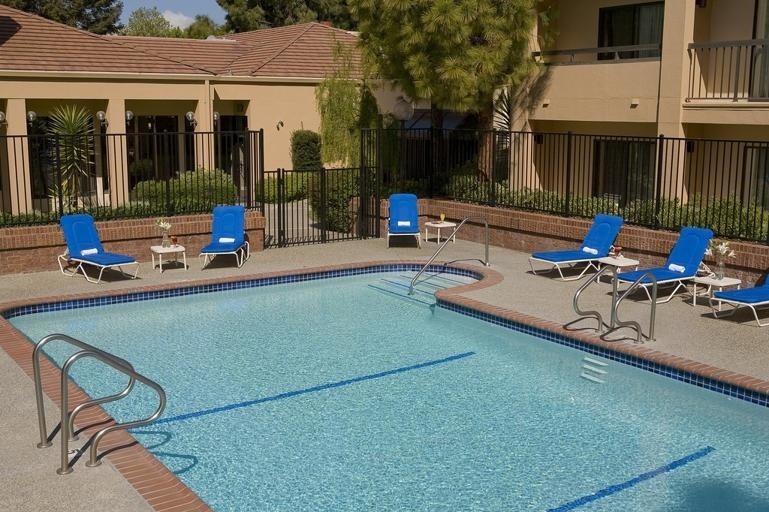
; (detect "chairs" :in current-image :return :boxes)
[617,227,715,304]
[709,272,769,327]
[386,193,422,249]
[527,213,625,281]
[57,214,139,284]
[198,205,250,270]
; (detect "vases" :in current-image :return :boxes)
[161,231,170,248]
[716,259,726,280]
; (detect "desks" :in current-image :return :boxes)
[424,221,457,245]
[150,244,187,273]
[693,277,742,312]
[597,256,640,289]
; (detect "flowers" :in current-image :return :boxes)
[703,236,737,261]
[154,214,172,232]
[607,239,623,259]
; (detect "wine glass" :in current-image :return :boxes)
[440,214,445,224]
[615,247,621,260]
[171,237,178,248]
